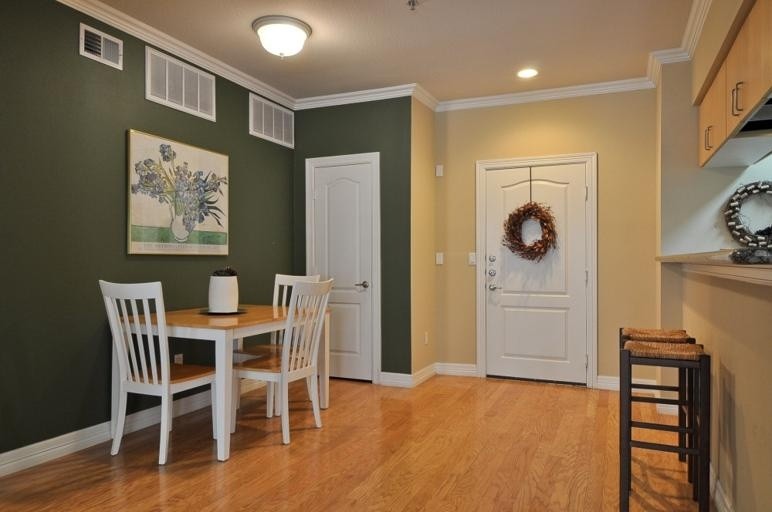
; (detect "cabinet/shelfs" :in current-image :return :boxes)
[727,6,770,145]
[697,59,724,169]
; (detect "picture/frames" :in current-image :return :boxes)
[125,126,229,259]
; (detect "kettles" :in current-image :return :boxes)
[208,265,239,314]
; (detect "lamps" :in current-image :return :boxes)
[251,14,313,61]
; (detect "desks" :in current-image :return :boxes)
[108,300,331,464]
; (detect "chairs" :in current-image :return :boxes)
[98,278,221,469]
[232,271,323,416]
[224,278,334,446]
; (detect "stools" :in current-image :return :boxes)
[621,341,715,512]
[621,329,698,489]
